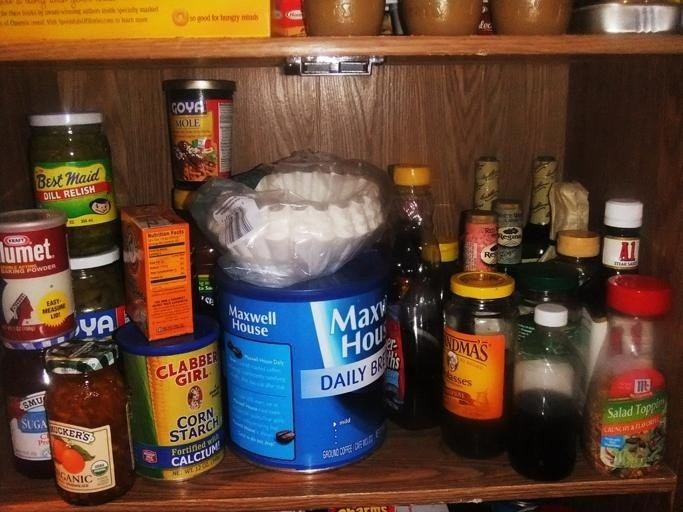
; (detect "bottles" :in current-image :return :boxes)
[560,230,602,311]
[462,207,500,271]
[582,194,645,383]
[496,198,524,271]
[589,273,671,478]
[473,157,496,208]
[123,206,195,337]
[392,164,439,437]
[516,261,570,334]
[445,272,510,460]
[425,237,458,277]
[513,303,579,480]
[545,180,586,239]
[529,152,559,240]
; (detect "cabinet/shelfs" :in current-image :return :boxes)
[0,0,680,507]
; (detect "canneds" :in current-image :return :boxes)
[174,193,213,240]
[78,247,126,331]
[31,110,114,251]
[119,312,228,481]
[210,266,389,473]
[0,207,75,342]
[46,344,134,506]
[164,79,234,189]
[194,243,218,302]
[4,344,58,482]
